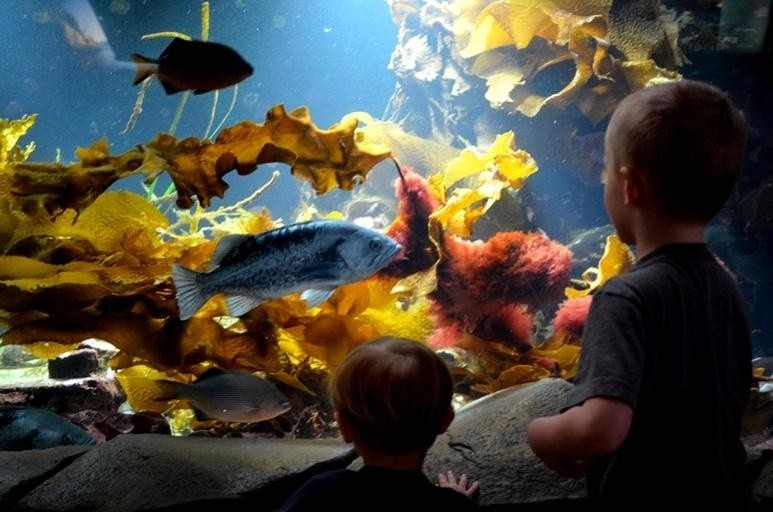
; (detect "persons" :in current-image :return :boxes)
[528,79,753,512]
[279,335,480,512]
